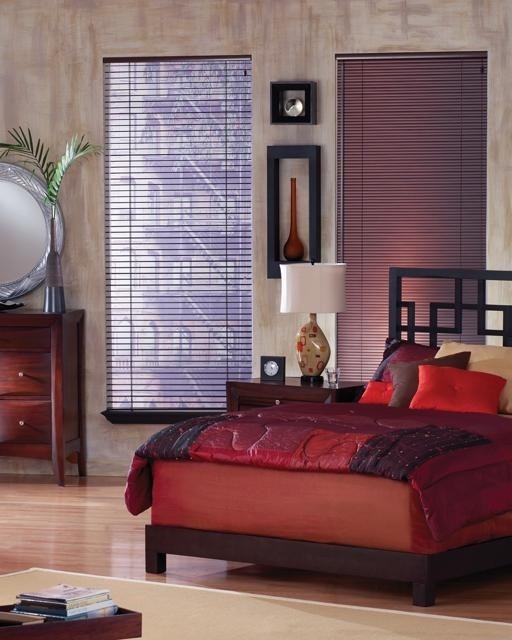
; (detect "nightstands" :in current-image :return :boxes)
[226,377,366,418]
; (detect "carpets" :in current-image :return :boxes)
[0,560,512,640]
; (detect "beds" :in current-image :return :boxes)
[121,266,512,607]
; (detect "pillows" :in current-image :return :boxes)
[358,376,396,405]
[383,348,470,410]
[372,335,439,385]
[410,357,505,420]
[432,340,512,416]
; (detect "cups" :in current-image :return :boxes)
[325,367,340,385]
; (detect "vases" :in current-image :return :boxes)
[281,175,309,263]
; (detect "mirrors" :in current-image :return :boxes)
[0,159,67,309]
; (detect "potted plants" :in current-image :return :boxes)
[0,126,112,313]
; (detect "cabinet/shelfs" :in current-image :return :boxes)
[0,305,89,484]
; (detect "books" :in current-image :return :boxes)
[0,581,120,628]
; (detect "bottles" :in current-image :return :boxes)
[283,178,304,260]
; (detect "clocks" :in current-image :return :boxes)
[259,353,288,384]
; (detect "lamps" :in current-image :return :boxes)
[276,262,347,384]
